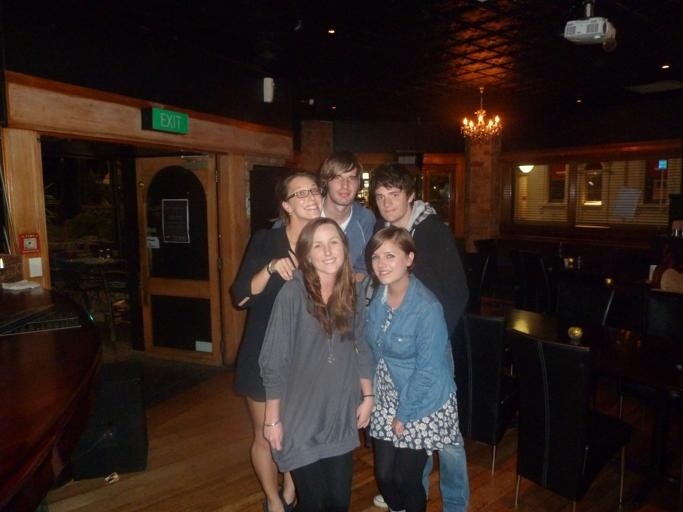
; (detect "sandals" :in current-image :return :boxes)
[281,489,297,512]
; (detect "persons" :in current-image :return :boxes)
[366,159,470,512]
[273,148,377,284]
[228,170,323,511]
[362,224,461,512]
[258,217,374,512]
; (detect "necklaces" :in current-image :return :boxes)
[325,329,337,365]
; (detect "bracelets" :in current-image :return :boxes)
[363,394,376,397]
[266,259,274,278]
[264,420,281,427]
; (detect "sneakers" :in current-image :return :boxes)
[373,493,389,508]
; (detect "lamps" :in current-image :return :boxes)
[459,87,502,144]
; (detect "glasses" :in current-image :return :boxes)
[286,187,323,201]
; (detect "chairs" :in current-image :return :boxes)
[460,236,605,332]
[505,329,631,511]
[615,289,682,507]
[449,313,522,476]
